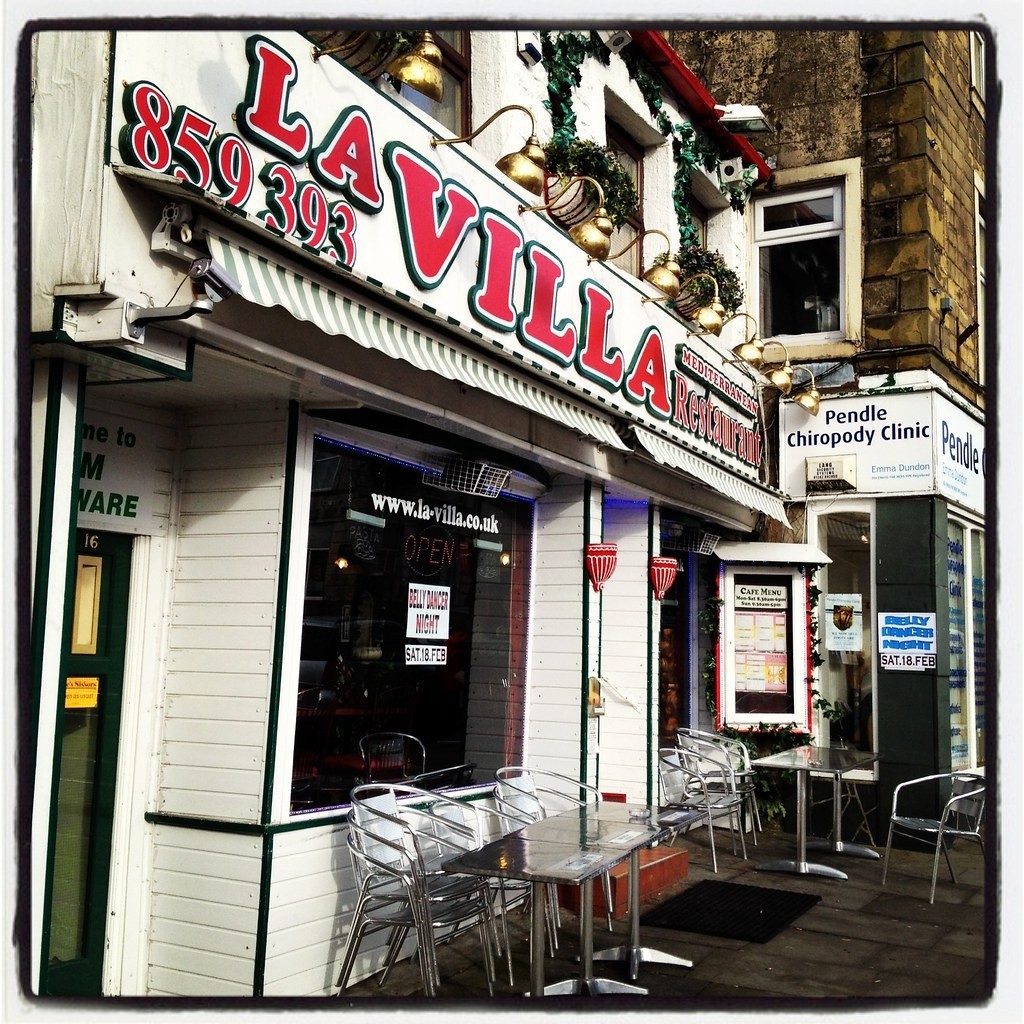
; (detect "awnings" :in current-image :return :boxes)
[148,190,802,533]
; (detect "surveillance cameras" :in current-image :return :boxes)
[188,257,242,303]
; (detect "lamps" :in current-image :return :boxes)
[430,105,547,197]
[722,340,792,395]
[313,28,444,103]
[640,273,726,337]
[687,313,764,371]
[713,103,774,135]
[752,366,820,417]
[589,229,680,296]
[518,177,612,262]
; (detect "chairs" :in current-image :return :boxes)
[333,782,496,997]
[658,747,747,874]
[289,675,476,813]
[493,766,613,957]
[674,727,764,856]
[882,772,987,904]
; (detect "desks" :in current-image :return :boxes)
[296,705,411,719]
[442,799,708,996]
[750,744,880,881]
